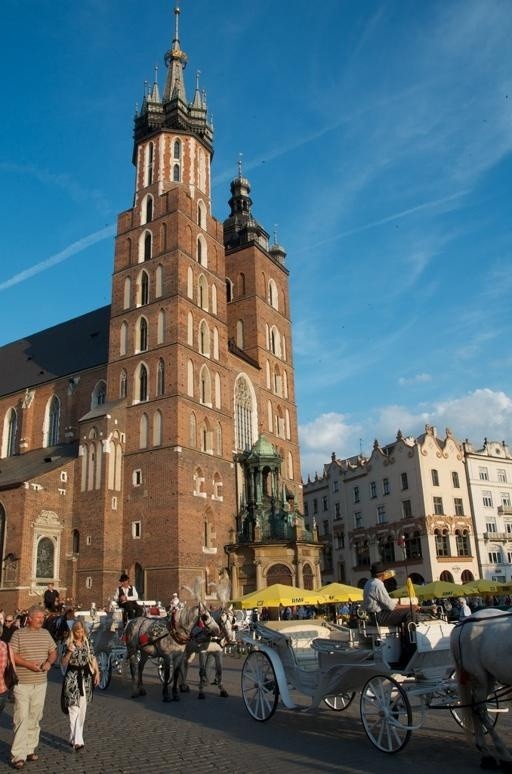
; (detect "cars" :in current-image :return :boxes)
[234,610,260,632]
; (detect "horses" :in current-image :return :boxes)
[172,603,236,699]
[119,602,221,702]
[41,607,76,669]
[450,607,512,774]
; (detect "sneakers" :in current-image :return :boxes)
[210,680,218,685]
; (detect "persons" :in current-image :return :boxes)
[115,575,143,618]
[223,615,248,654]
[57,608,77,677]
[0,610,20,713]
[363,563,421,630]
[252,604,353,623]
[59,620,100,752]
[8,605,58,768]
[43,582,62,614]
[170,593,179,607]
[458,597,471,617]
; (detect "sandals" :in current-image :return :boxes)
[26,752,39,761]
[68,741,84,753]
[13,759,25,769]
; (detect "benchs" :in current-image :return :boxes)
[109,601,163,624]
[256,618,349,658]
[74,611,108,629]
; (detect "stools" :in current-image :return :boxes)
[358,611,400,638]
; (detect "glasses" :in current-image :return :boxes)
[4,619,13,623]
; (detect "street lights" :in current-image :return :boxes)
[399,535,408,578]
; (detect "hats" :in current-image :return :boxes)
[368,561,389,575]
[119,574,130,583]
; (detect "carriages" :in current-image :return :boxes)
[60,599,238,702]
[18,602,72,648]
[236,608,510,771]
